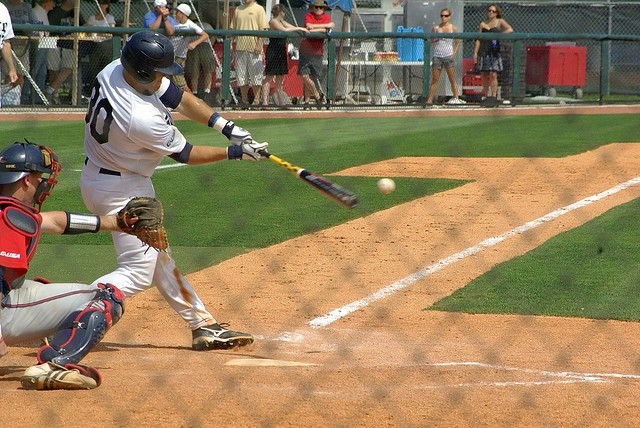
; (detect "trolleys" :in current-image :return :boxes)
[524,45,586,97]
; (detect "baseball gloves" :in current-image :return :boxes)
[115,195,168,255]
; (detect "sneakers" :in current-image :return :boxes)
[192,322,254,351]
[46,92,53,104]
[19,361,97,390]
[53,92,62,106]
[303,102,311,110]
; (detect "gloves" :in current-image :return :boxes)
[222,119,252,144]
[226,141,268,162]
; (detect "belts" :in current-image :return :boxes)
[84,157,121,177]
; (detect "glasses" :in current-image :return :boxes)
[441,14,450,17]
[488,9,497,13]
[315,6,323,9]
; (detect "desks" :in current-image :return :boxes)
[321,61,425,105]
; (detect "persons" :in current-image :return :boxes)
[143,0,176,36]
[299,0,336,110]
[423,6,461,106]
[78,30,266,348]
[189,21,215,105]
[171,4,209,96]
[82,1,118,95]
[262,0,309,111]
[472,4,515,103]
[1,0,19,84]
[23,0,58,105]
[1,0,32,87]
[48,1,86,106]
[228,0,270,110]
[0,140,138,390]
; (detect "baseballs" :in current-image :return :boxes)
[377,177,396,195]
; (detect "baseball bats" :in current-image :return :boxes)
[244,139,359,210]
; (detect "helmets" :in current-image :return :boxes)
[1,138,61,207]
[119,30,184,86]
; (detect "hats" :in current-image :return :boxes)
[309,0,328,10]
[154,0,168,8]
[170,3,192,17]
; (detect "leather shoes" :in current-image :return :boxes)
[316,93,324,110]
